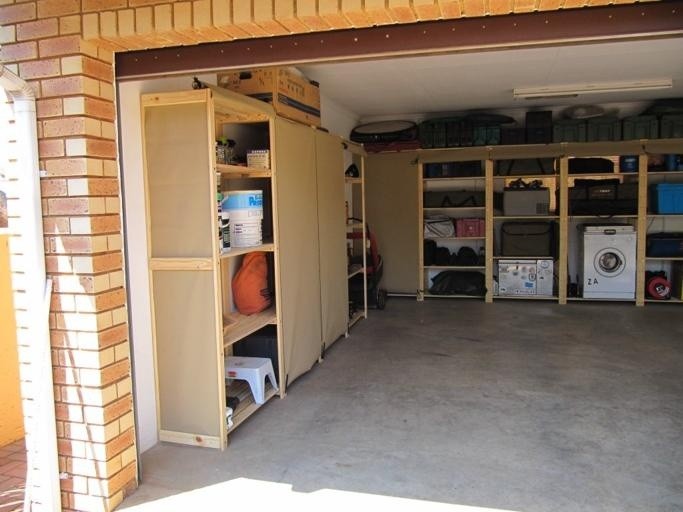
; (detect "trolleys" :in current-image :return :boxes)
[346,217,388,309]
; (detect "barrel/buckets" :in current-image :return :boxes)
[221,191,264,247]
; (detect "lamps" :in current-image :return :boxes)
[513,78,674,100]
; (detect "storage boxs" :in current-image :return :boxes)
[649,183,683,215]
[215,67,321,127]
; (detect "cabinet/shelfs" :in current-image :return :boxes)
[140,89,322,452]
[417,138,683,308]
[316,129,367,352]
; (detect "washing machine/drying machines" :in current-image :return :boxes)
[583,225,637,300]
